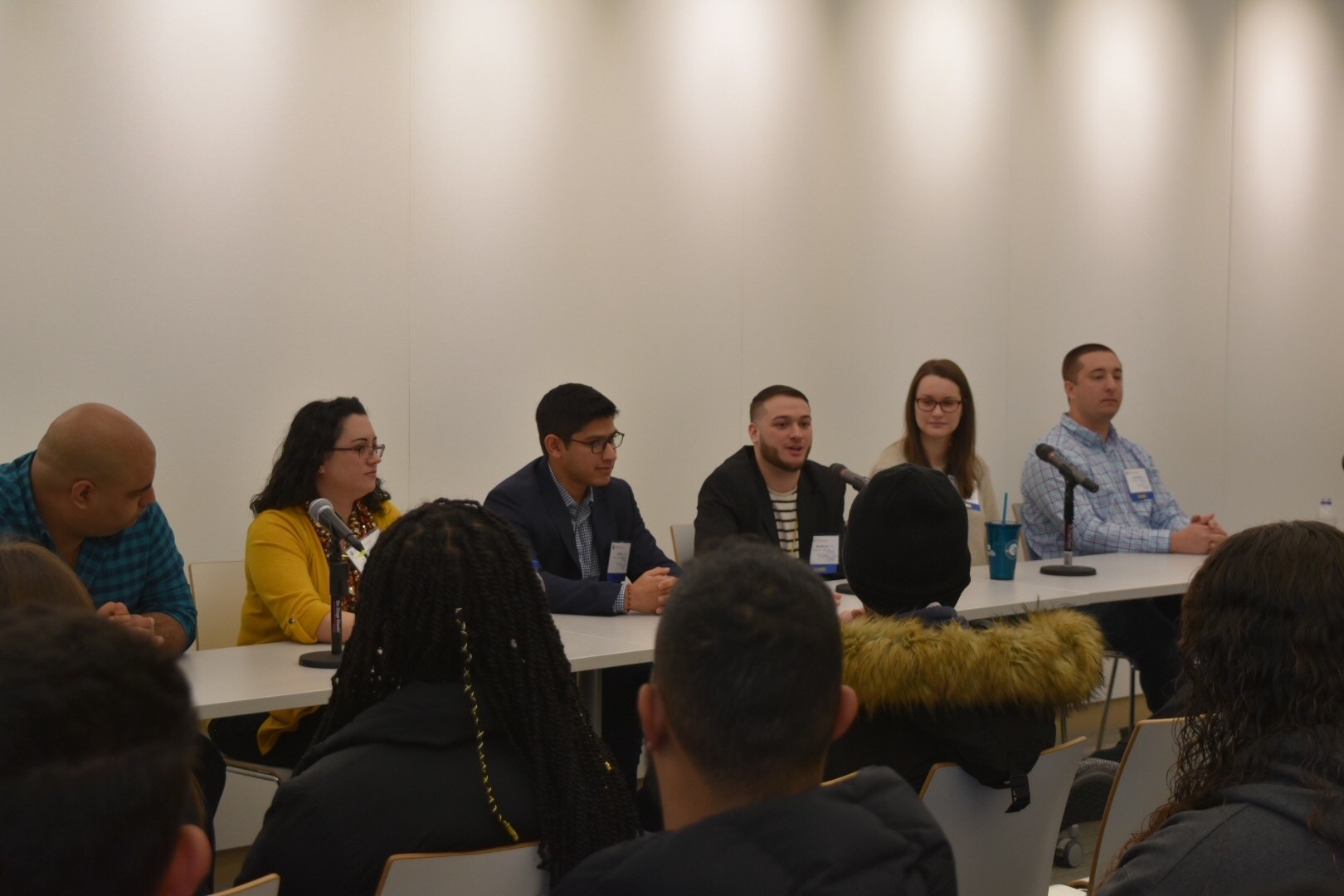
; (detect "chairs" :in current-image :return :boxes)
[917,736,1086,896]
[669,522,697,571]
[187,559,552,896]
[1069,713,1224,896]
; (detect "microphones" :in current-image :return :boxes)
[309,498,365,554]
[1036,443,1099,493]
[829,463,868,492]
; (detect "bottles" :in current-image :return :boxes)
[1315,498,1337,528]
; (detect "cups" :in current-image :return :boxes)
[985,520,1022,581]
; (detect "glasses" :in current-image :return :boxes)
[569,430,624,453]
[914,397,966,413]
[334,445,386,460]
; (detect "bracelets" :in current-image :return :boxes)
[626,584,631,613]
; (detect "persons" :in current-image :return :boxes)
[1020,344,1227,716]
[480,383,682,821]
[0,465,1344,896]
[688,384,854,582]
[870,359,1002,565]
[0,402,199,664]
[226,396,402,767]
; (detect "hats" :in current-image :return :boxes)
[843,463,971,611]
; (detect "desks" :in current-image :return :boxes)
[550,552,1208,737]
